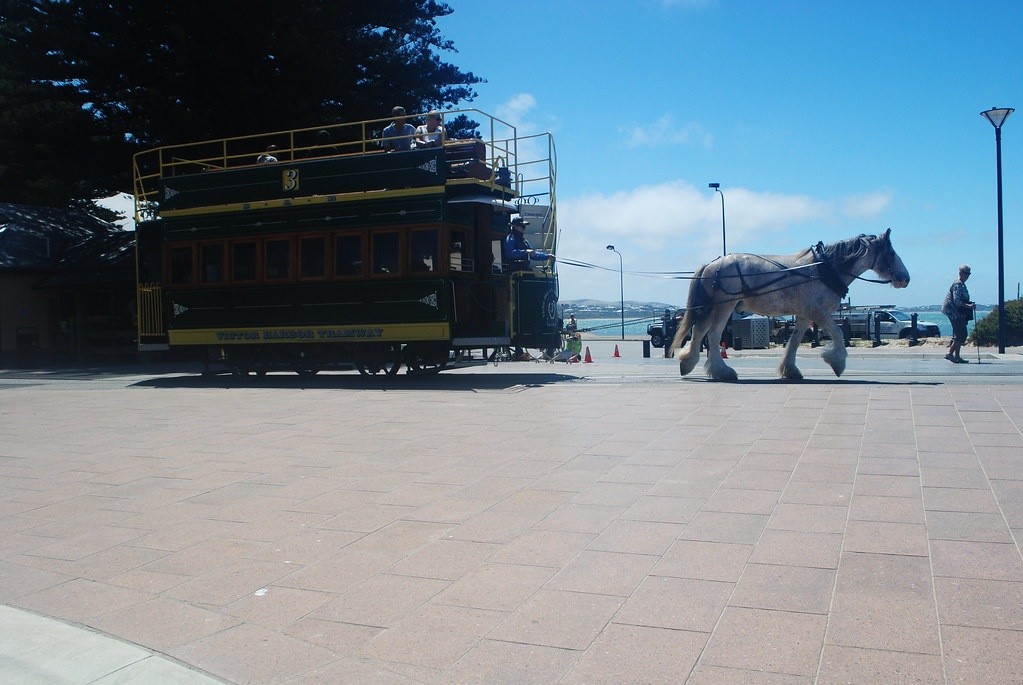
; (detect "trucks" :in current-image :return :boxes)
[808,297,940,340]
[646,308,733,346]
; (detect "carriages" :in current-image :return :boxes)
[132,108,910,380]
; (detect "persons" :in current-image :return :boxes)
[504,217,556,276]
[942,264,976,363]
[566,333,582,362]
[414,110,447,149]
[566,313,577,337]
[257,145,279,164]
[416,253,429,271]
[382,107,416,152]
[884,313,892,321]
[310,130,337,157]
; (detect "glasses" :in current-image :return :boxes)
[518,225,525,229]
[434,117,442,121]
[961,271,971,275]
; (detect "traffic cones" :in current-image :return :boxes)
[614,345,619,357]
[584,346,592,362]
[720,342,727,358]
[570,345,579,362]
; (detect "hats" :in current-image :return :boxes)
[512,217,530,225]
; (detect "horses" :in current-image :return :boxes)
[666,226,912,383]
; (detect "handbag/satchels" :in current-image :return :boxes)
[966,302,973,320]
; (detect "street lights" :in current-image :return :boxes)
[982,107,1014,354]
[708,183,726,256]
[606,245,624,339]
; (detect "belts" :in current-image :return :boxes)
[514,260,527,262]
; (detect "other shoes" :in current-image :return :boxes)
[954,357,969,363]
[944,354,959,363]
